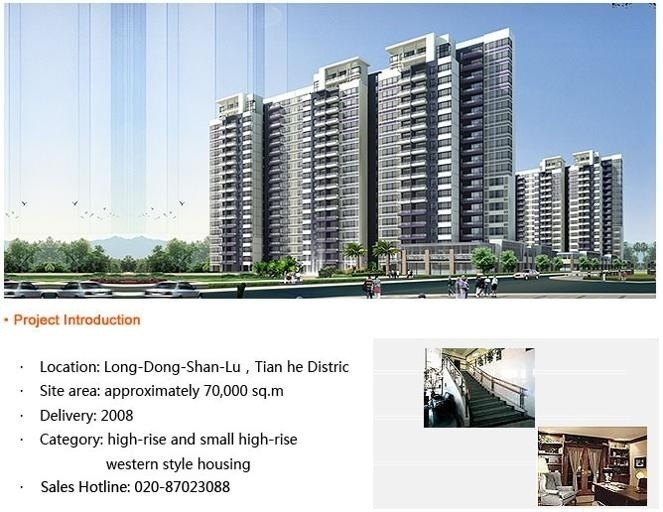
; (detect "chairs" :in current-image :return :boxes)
[638,478,647,487]
[540,472,577,506]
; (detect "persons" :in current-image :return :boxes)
[407,269,413,279]
[619,270,627,280]
[447,275,499,298]
[284,269,304,284]
[365,274,381,299]
[388,269,399,279]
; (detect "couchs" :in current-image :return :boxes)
[431,391,456,427]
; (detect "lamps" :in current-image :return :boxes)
[538,457,549,477]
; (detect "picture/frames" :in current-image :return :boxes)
[634,457,646,468]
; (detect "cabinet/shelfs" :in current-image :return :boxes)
[538,431,565,475]
[609,447,630,485]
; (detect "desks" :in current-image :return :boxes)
[591,481,647,506]
